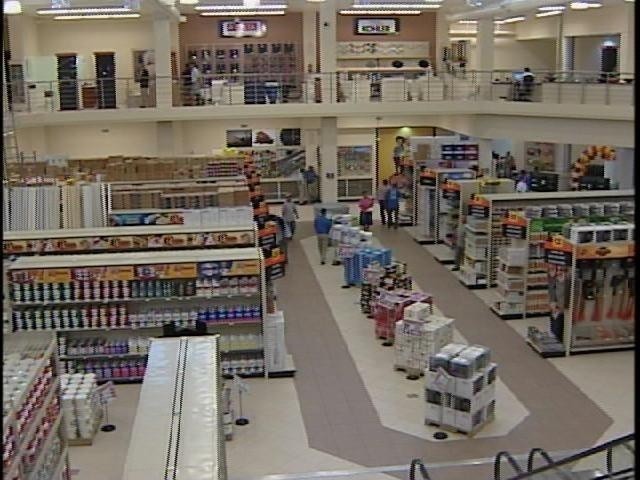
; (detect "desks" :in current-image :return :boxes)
[339,72,473,102]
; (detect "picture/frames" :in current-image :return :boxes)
[218,20,268,38]
[353,16,400,35]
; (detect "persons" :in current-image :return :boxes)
[313,208,333,266]
[384,180,411,229]
[297,168,308,206]
[179,63,194,106]
[390,136,405,177]
[501,150,531,192]
[515,66,536,90]
[377,179,393,226]
[357,190,375,233]
[281,193,300,242]
[187,60,206,106]
[302,165,321,203]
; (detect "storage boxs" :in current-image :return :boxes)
[326,215,495,437]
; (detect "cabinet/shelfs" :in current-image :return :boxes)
[2,176,267,480]
[414,166,635,358]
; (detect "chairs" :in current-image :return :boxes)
[513,72,534,100]
